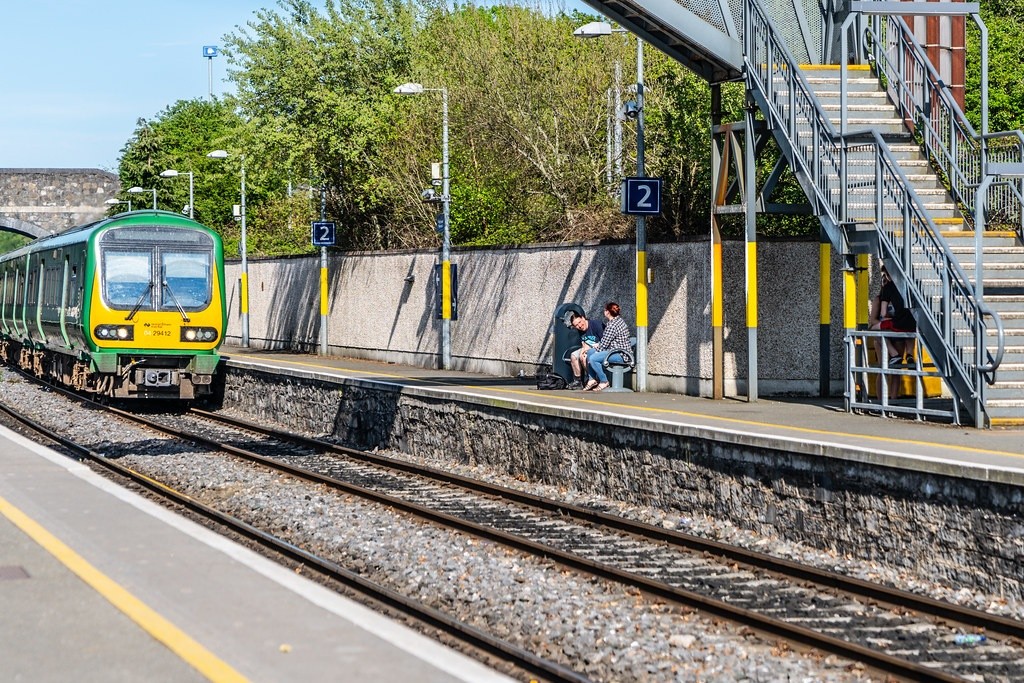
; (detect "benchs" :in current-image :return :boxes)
[562,337,636,393]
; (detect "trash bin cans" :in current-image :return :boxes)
[553,304,585,384]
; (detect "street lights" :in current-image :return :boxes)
[160,169,194,218]
[205,149,250,349]
[126,186,157,210]
[393,81,451,371]
[572,21,650,393]
[104,198,132,211]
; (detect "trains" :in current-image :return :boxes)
[0,209,230,412]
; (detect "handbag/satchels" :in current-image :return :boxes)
[534,374,567,390]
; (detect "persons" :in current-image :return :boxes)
[565,313,606,389]
[582,303,634,391]
[867,266,916,400]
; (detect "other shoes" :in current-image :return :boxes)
[905,355,915,370]
[889,354,903,365]
[583,381,597,390]
[567,381,583,389]
[593,381,610,392]
